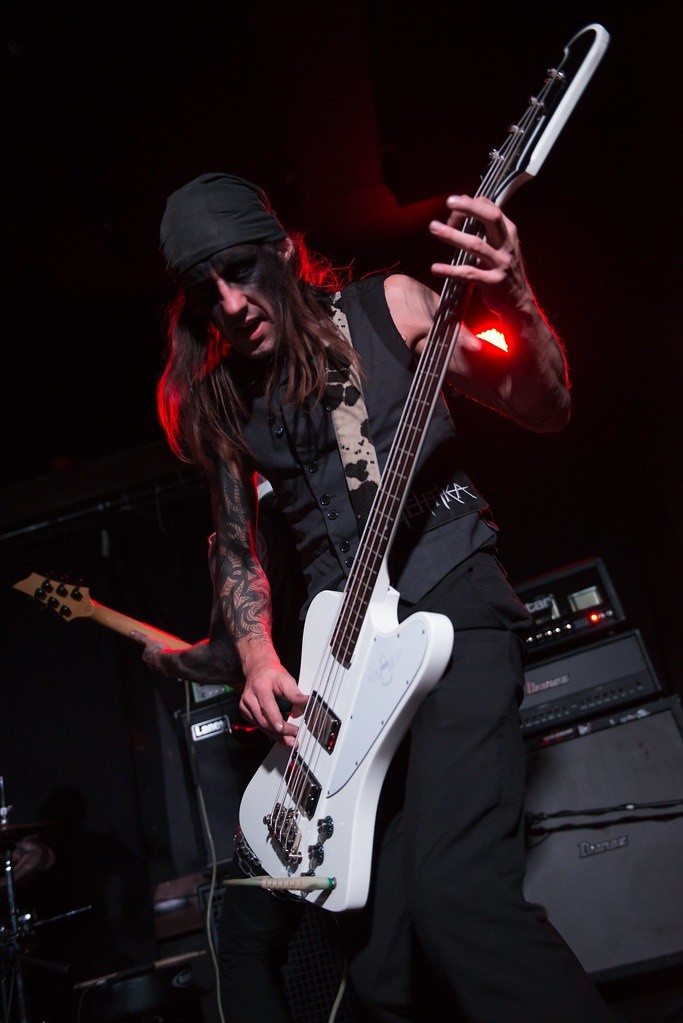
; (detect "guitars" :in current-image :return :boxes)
[238,20,611,916]
[13,570,193,651]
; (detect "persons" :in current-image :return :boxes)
[0,775,55,1023]
[142,519,307,1023]
[157,173,621,1023]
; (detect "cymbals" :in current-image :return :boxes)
[0,819,69,847]
